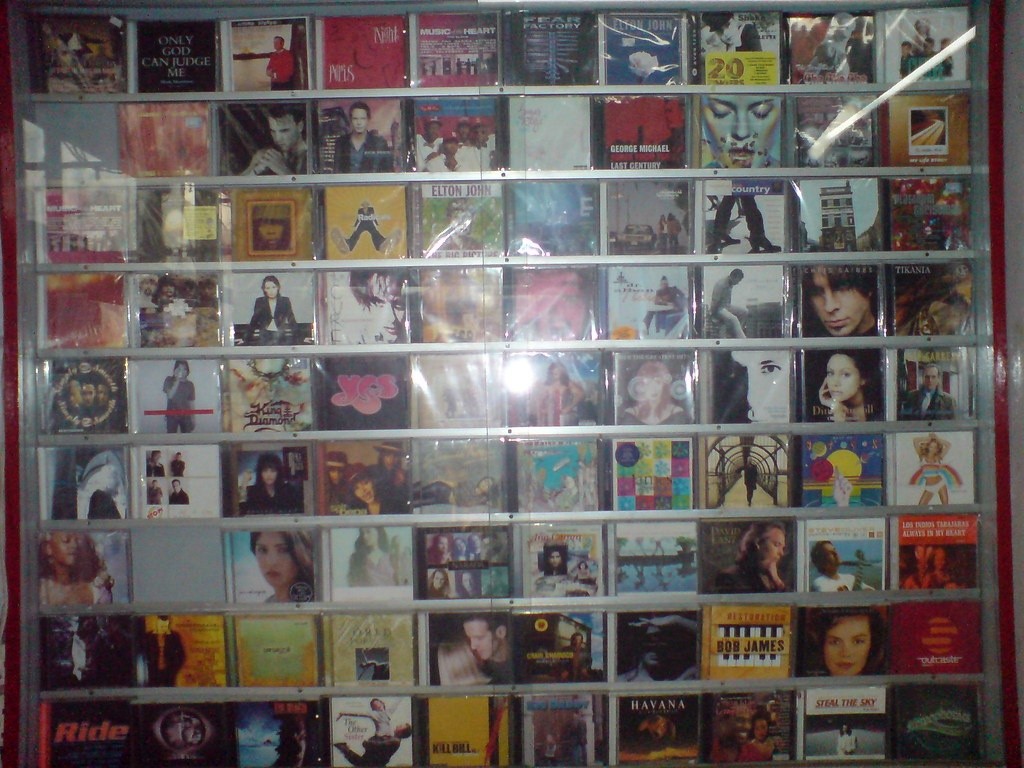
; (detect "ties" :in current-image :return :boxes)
[921,393,930,418]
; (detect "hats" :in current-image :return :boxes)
[325,452,346,471]
[372,440,404,455]
[339,464,373,493]
[252,206,288,221]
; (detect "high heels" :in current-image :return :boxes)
[749,231,781,253]
[713,230,740,246]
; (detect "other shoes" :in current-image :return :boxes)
[380,229,402,256]
[330,228,349,254]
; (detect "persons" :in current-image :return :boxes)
[14,7,977,766]
